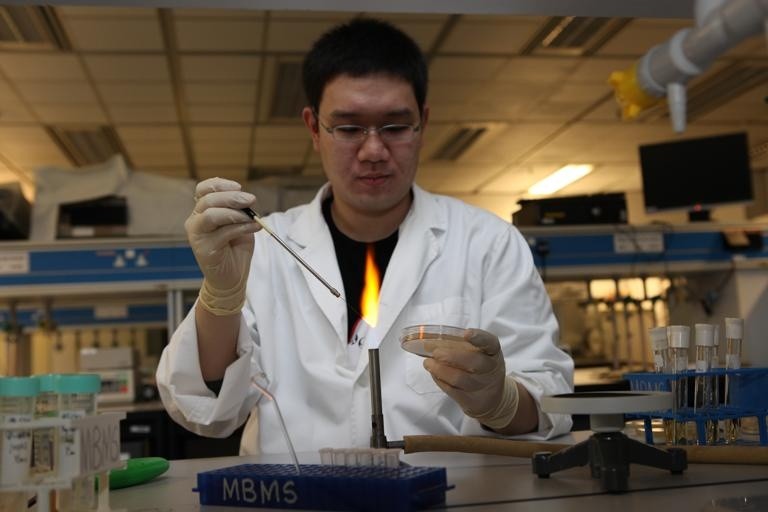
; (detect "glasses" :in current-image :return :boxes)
[316,119,423,145]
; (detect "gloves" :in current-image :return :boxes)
[421,326,520,431]
[184,178,262,316]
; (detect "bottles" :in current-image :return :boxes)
[651,319,744,451]
[318,448,399,470]
[0,373,112,512]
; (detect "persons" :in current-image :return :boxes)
[154,16,574,460]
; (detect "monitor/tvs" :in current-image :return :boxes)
[638,131,754,214]
